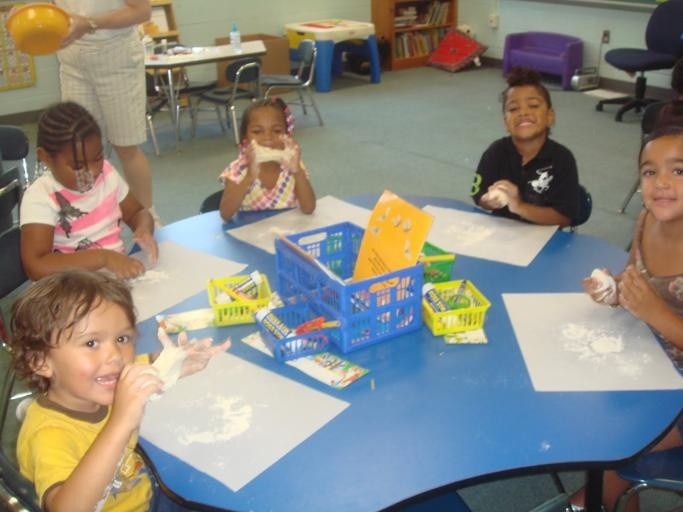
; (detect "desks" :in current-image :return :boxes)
[126,193,680,512]
[282,17,381,93]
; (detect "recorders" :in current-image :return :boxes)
[569,67,600,90]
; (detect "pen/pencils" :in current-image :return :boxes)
[310,320,341,329]
[418,254,456,262]
[295,317,325,334]
[454,279,467,303]
[211,278,258,312]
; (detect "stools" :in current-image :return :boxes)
[613,447,681,512]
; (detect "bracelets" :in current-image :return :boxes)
[88,17,97,35]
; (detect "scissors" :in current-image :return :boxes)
[446,295,471,310]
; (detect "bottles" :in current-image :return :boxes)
[144,36,193,61]
[217,271,451,349]
[229,21,242,51]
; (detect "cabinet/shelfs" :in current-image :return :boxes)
[145,2,188,108]
[370,0,457,71]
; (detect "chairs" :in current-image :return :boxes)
[0,449,40,512]
[595,0,682,122]
[144,32,323,156]
[0,222,30,434]
[614,100,683,217]
[503,30,582,90]
[197,189,222,214]
[1,123,31,186]
[0,165,23,226]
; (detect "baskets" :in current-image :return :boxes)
[273,222,425,355]
[206,272,273,327]
[420,239,456,282]
[421,278,491,337]
[253,304,333,361]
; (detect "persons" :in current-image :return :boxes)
[10,266,232,512]
[471,65,578,229]
[217,96,316,221]
[18,101,158,281]
[566,100,683,512]
[53,0,161,227]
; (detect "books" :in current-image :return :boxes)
[394,0,450,58]
